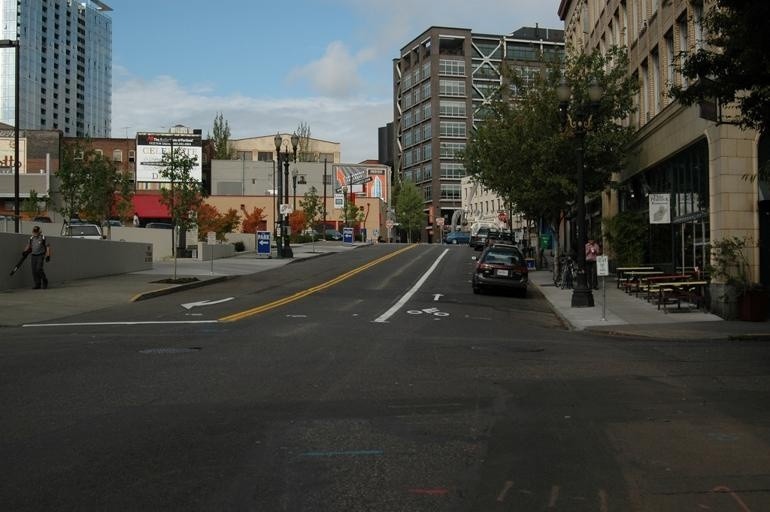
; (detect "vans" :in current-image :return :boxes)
[145,222,175,229]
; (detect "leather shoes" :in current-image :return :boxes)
[43,279,48,288]
[33,285,41,288]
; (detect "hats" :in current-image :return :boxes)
[32,226,42,234]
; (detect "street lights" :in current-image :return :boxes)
[0,40,20,233]
[554,73,604,308]
[274,131,299,258]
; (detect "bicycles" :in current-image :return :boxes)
[532,247,548,268]
[554,256,575,290]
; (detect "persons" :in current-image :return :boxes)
[22,226,51,289]
[133,212,139,228]
[585,236,600,290]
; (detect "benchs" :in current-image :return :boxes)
[616,265,710,314]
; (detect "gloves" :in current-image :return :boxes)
[45,256,50,262]
[22,251,27,256]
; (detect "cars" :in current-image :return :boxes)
[442,227,528,296]
[33,215,122,240]
[300,229,343,241]
